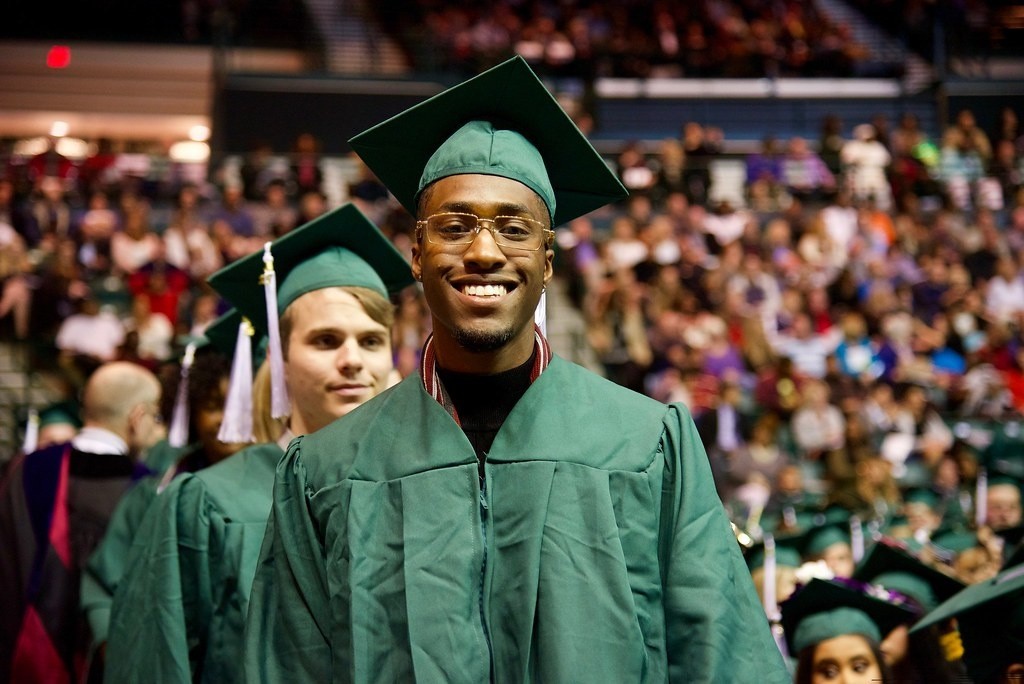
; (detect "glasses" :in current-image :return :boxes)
[414,211,557,251]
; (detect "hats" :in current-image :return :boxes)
[849,540,968,613]
[907,563,1024,634]
[205,202,415,418]
[728,409,1023,575]
[204,307,264,446]
[348,54,629,229]
[780,578,916,656]
[24,397,79,454]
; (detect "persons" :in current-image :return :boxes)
[0,361,166,684]
[103,202,417,684]
[419,0,872,57]
[82,306,289,664]
[1,109,1023,610]
[777,577,915,684]
[238,57,793,684]
[847,539,966,684]
[907,576,1023,684]
